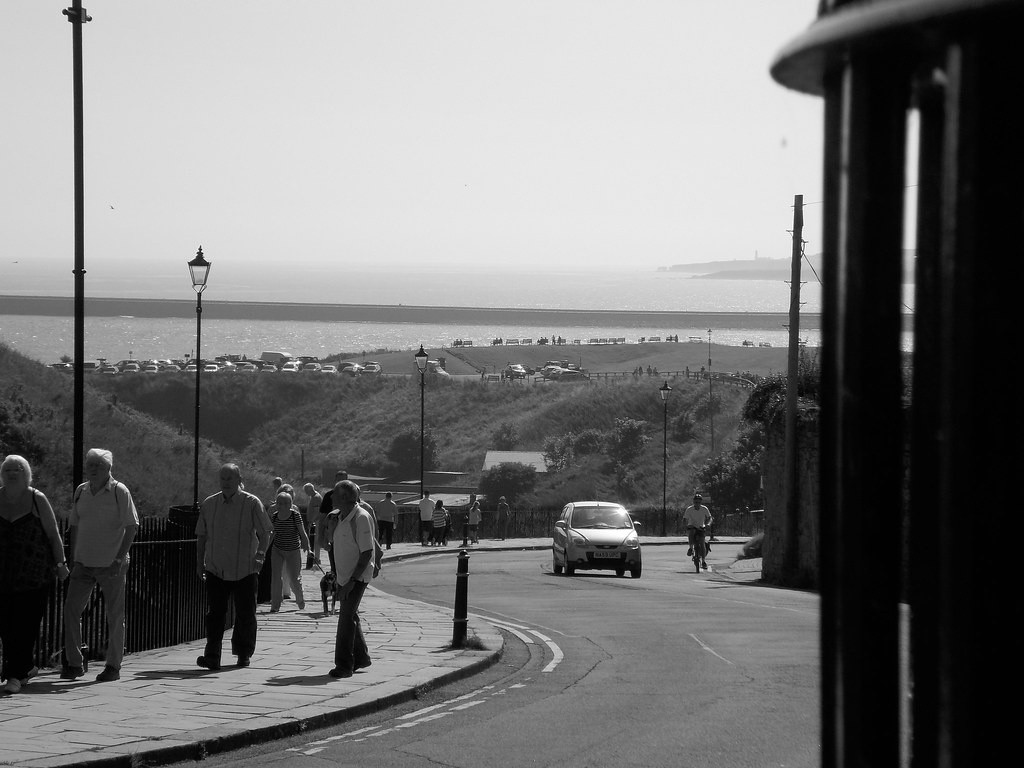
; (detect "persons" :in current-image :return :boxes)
[0,455,69,693]
[195,461,511,677]
[61,448,140,682]
[682,494,712,569]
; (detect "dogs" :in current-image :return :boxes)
[319,571,340,617]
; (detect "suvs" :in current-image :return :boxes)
[337,361,382,377]
[504,364,535,379]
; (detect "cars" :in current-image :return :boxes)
[242,364,258,373]
[281,360,303,373]
[552,501,642,578]
[321,365,337,376]
[260,365,278,373]
[204,365,220,372]
[234,351,320,367]
[186,365,198,372]
[302,363,321,373]
[53,359,181,375]
[536,360,579,377]
[223,365,238,373]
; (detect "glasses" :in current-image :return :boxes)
[693,494,700,498]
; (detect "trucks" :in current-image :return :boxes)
[413,361,449,378]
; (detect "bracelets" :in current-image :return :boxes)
[255,559,263,564]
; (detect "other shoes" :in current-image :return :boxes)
[298,601,305,610]
[96,665,120,681]
[59,667,84,680]
[270,609,280,613]
[329,665,353,678]
[702,562,708,569]
[237,655,250,666]
[687,548,694,556]
[353,653,372,674]
[197,655,221,670]
[5,666,38,692]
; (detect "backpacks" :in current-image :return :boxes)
[350,512,383,578]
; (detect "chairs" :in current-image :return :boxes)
[602,510,618,527]
[573,510,588,526]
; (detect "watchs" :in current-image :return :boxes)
[114,558,123,563]
[349,576,356,582]
[57,561,68,567]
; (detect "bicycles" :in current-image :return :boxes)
[685,525,707,573]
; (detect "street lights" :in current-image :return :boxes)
[659,381,672,535]
[707,328,712,370]
[186,246,211,535]
[414,344,429,499]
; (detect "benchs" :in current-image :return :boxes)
[586,337,625,345]
[572,339,581,345]
[741,341,772,348]
[647,337,661,342]
[665,337,675,342]
[689,336,703,342]
[638,336,645,343]
[537,339,548,346]
[520,339,532,345]
[798,341,807,347]
[493,340,503,346]
[555,339,567,345]
[506,338,519,346]
[485,374,500,382]
[454,341,472,347]
